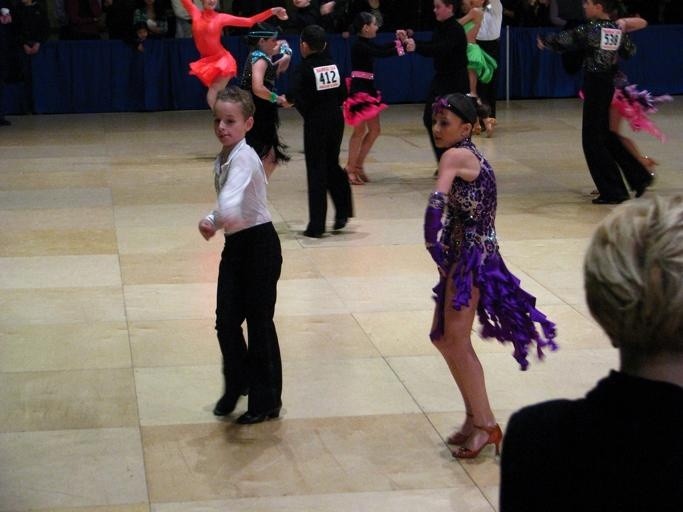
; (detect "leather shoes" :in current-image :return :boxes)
[591,177,652,204]
[234,400,282,424]
[214,385,251,415]
[300,218,348,237]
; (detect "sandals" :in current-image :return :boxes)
[344,163,369,185]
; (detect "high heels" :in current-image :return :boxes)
[446,411,503,460]
[473,117,496,137]
[643,155,659,180]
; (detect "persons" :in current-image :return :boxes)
[180,0,503,186]
[199,85,283,425]
[499,195,683,512]
[538,1,676,205]
[422,93,502,459]
[286,24,353,237]
[1,1,12,126]
[13,2,190,54]
